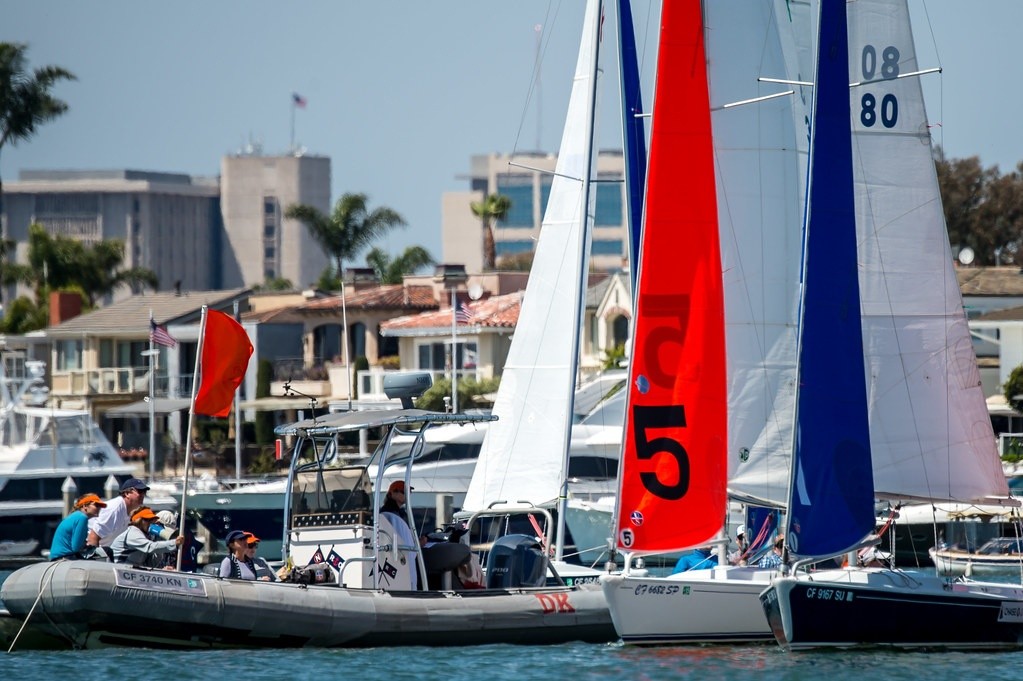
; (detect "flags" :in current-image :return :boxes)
[194,308,254,417]
[294,96,306,108]
[456,297,472,324]
[150,322,176,348]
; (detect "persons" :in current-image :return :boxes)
[759,534,784,569]
[219,530,282,582]
[86,478,150,548]
[382,481,427,546]
[727,525,744,562]
[49,493,107,560]
[672,546,718,574]
[109,506,184,570]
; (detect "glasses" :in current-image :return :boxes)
[737,534,744,539]
[396,489,411,495]
[86,503,101,509]
[773,543,782,549]
[246,543,259,548]
[132,489,147,495]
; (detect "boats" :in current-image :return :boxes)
[561,480,756,567]
[169,283,787,577]
[929,511,1023,577]
[0,538,39,556]
[870,496,1023,564]
[0,343,142,553]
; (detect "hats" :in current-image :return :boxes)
[225,531,252,546]
[120,479,150,492]
[242,531,260,543]
[736,524,746,534]
[774,534,785,544]
[389,480,414,491]
[132,509,160,521]
[75,494,107,508]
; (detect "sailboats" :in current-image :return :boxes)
[0,0,1023,652]
